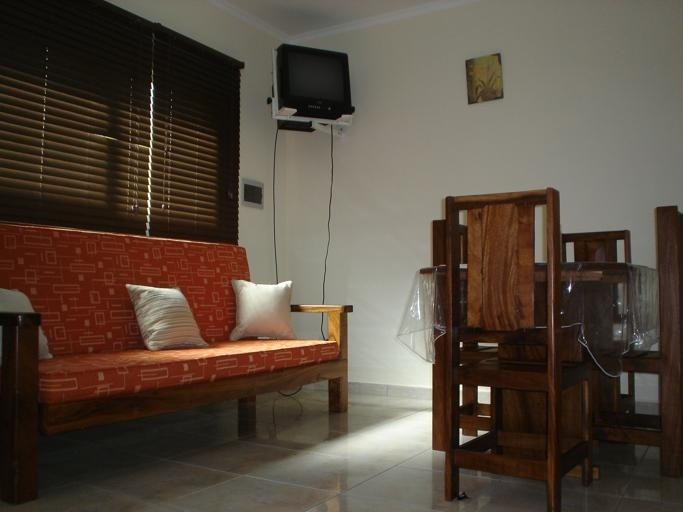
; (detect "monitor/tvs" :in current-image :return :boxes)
[276,43,355,119]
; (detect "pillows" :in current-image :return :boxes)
[227,275,298,342]
[122,278,209,355]
[0,283,54,364]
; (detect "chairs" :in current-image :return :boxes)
[544,224,631,414]
[428,214,495,453]
[439,179,598,511]
[579,198,682,486]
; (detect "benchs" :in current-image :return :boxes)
[0,216,353,507]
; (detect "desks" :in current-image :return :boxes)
[414,261,661,486]
